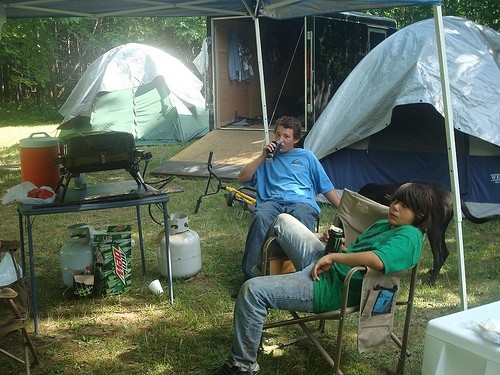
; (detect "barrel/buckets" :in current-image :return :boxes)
[20,132,61,191]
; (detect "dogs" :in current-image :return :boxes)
[338,182,455,286]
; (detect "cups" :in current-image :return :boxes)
[148,279,164,295]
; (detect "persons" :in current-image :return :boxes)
[216,182,437,375]
[230,115,341,300]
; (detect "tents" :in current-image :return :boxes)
[57,43,209,145]
[0,1,500,314]
[291,15,500,225]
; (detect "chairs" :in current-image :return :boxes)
[256,189,426,375]
[0,239,40,375]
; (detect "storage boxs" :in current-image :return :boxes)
[63,268,95,299]
[421,300,500,375]
[92,225,133,297]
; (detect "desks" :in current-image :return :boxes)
[17,181,173,334]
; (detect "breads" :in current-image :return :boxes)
[28,188,53,198]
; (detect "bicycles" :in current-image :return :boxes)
[193,150,258,216]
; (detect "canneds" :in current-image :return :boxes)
[266,140,281,161]
[324,227,343,255]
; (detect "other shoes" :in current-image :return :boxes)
[216,359,260,375]
[230,283,243,302]
